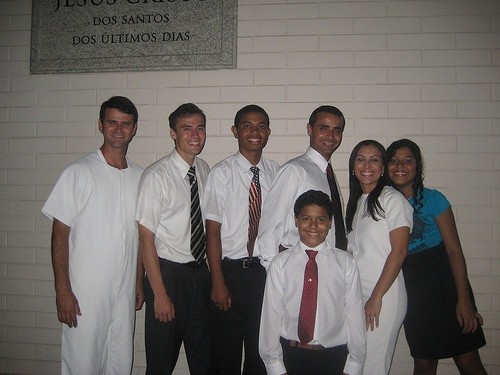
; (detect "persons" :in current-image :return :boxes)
[202,104,282,375]
[41,96,147,375]
[385,139,491,375]
[258,189,367,375]
[342,140,413,375]
[260,105,347,273]
[134,102,212,375]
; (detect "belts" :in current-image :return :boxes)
[222,258,258,270]
[286,340,345,353]
[158,256,207,267]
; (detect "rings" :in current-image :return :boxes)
[369,318,374,321]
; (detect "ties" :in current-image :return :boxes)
[326,163,348,251]
[246,166,262,261]
[297,250,319,345]
[187,167,207,265]
